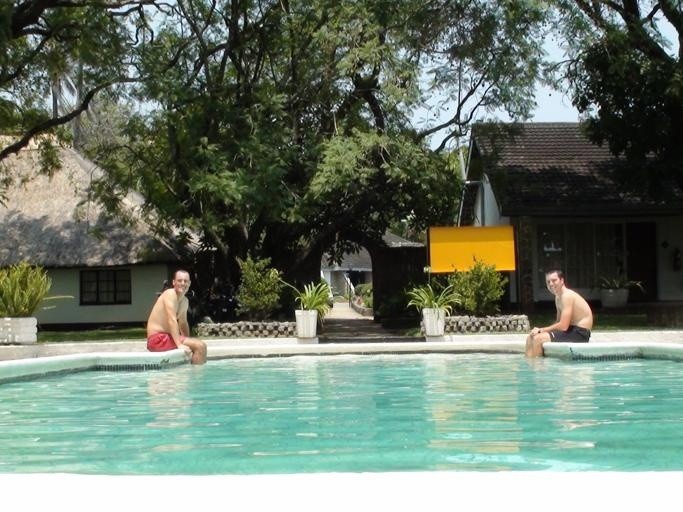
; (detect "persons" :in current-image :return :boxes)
[147,270,208,366]
[525,268,594,358]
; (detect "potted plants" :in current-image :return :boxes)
[405,282,463,337]
[598,267,647,309]
[275,268,330,339]
[0,258,76,344]
